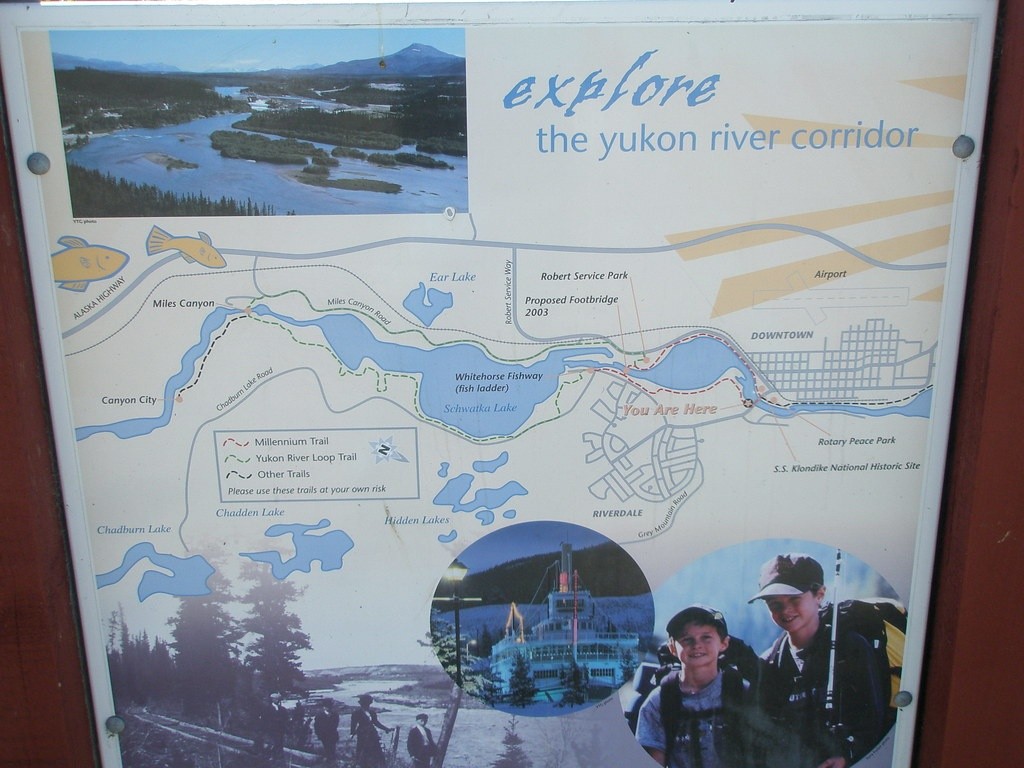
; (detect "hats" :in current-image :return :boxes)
[666,605,725,636]
[358,695,373,705]
[748,553,825,602]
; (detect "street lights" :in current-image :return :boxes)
[465,639,477,667]
[446,558,470,690]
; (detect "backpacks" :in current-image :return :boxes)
[771,600,891,719]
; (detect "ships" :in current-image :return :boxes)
[488,540,641,699]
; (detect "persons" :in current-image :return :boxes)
[637,606,751,768]
[751,552,908,768]
[260,693,441,768]
